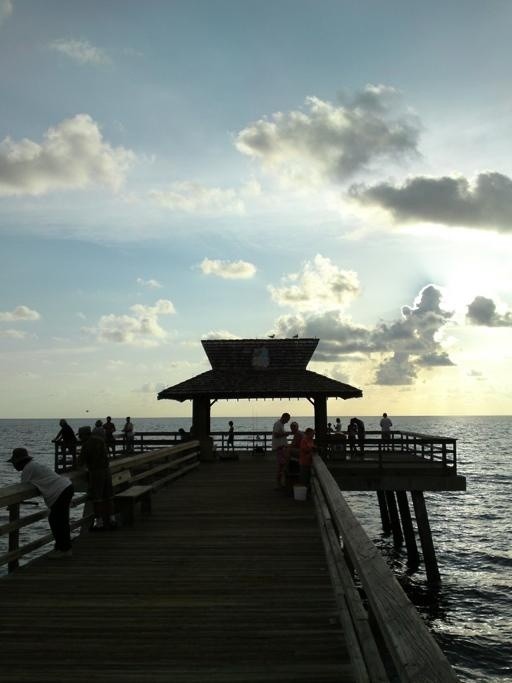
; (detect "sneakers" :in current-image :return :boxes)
[46,547,73,559]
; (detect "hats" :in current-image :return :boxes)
[6,448,35,463]
[75,426,93,436]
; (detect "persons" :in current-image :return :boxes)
[179,427,190,441]
[92,419,105,440]
[224,419,235,452]
[378,412,393,451]
[73,426,120,533]
[53,418,79,462]
[7,447,77,560]
[102,416,117,459]
[268,409,368,494]
[122,417,135,453]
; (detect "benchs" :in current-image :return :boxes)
[111,469,153,523]
